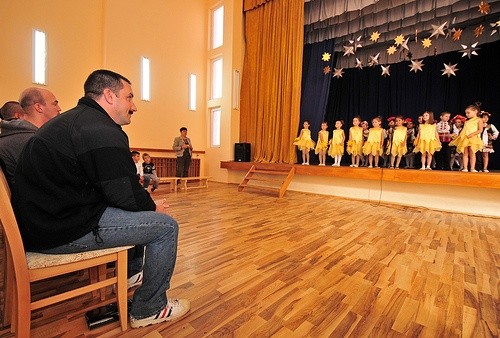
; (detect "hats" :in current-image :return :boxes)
[450,114,467,124]
[478,111,491,116]
[405,118,415,123]
[361,121,368,126]
[387,117,396,122]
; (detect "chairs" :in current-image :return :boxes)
[0,166,135,338]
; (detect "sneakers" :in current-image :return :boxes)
[129,298,190,329]
[112,270,144,294]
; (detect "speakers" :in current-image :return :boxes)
[235,143,251,162]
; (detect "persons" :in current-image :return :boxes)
[449,103,484,172]
[0,87,61,181]
[479,112,499,172]
[131,151,159,192]
[413,111,442,170]
[363,116,396,168]
[434,111,467,172]
[328,120,345,166]
[347,116,369,167]
[173,127,193,186]
[11,69,190,327]
[315,121,329,166]
[293,121,315,165]
[387,116,417,169]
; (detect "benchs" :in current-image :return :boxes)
[153,176,210,194]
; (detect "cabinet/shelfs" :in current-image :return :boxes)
[152,158,201,179]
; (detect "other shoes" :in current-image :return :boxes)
[479,169,489,173]
[368,165,380,169]
[405,165,415,168]
[450,165,463,171]
[302,163,309,166]
[361,164,368,166]
[332,163,340,167]
[151,188,155,192]
[420,167,431,170]
[350,164,358,168]
[431,161,436,168]
[389,166,399,170]
[462,168,478,173]
[319,164,325,167]
